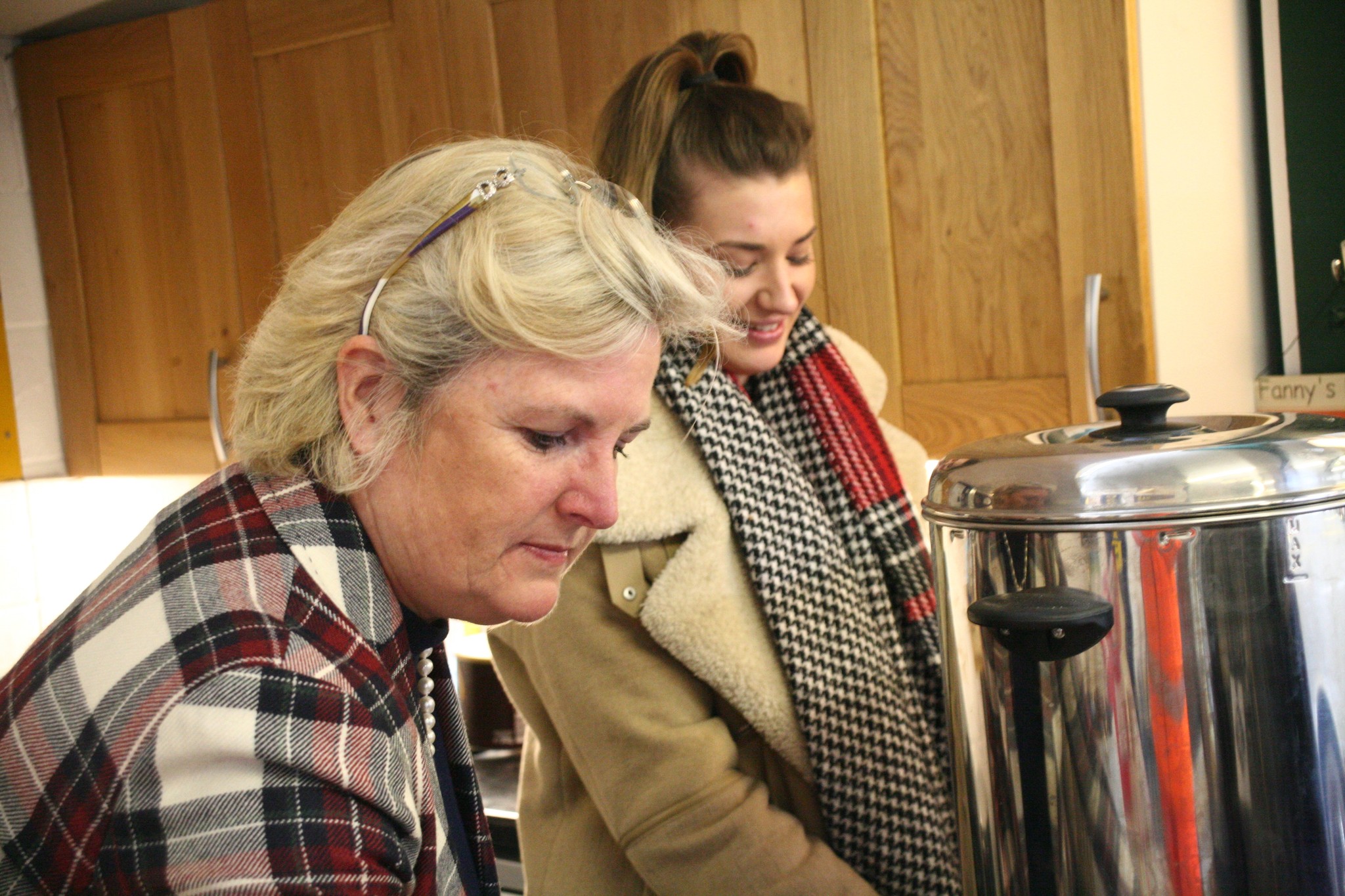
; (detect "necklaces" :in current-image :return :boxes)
[1003,532,1029,591]
[417,648,436,760]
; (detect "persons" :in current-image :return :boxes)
[3,138,751,896]
[490,31,958,896]
[953,486,1158,896]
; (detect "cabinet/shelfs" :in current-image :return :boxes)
[6,2,1160,479]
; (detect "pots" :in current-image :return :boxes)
[919,384,1345,896]
